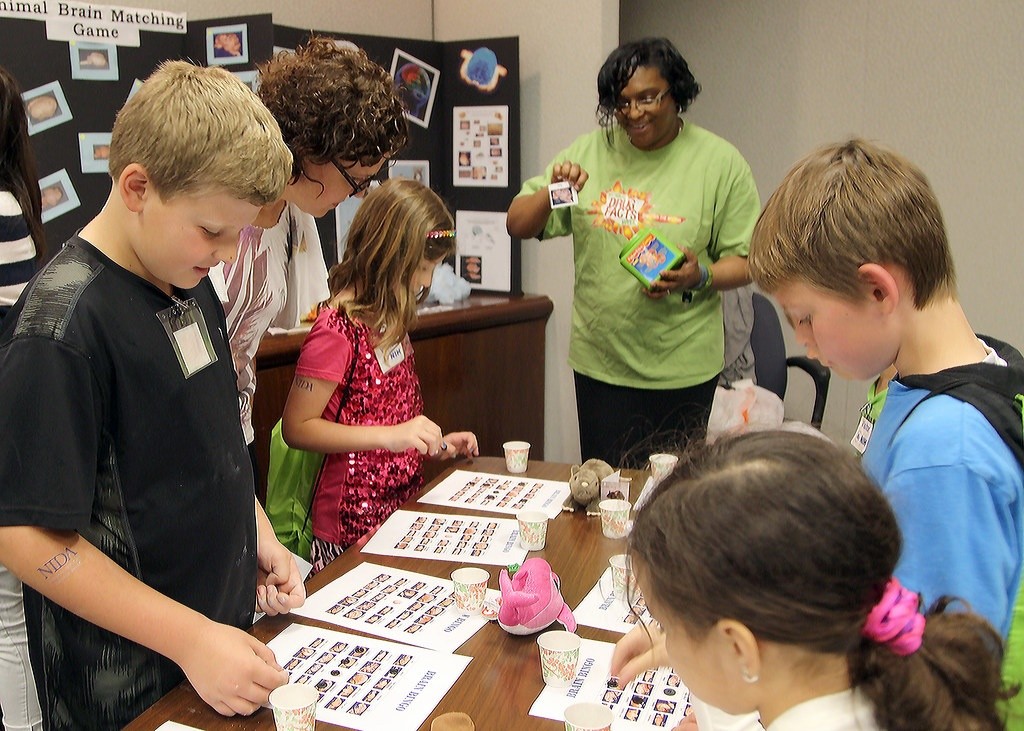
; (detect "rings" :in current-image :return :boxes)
[666,288,671,296]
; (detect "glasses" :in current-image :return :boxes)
[330,160,376,198]
[614,88,671,115]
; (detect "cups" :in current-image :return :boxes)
[503,441,531,473]
[537,631,581,687]
[608,554,641,601]
[649,454,678,485]
[451,567,491,616]
[564,702,615,731]
[599,499,632,539]
[268,683,318,731]
[516,510,548,551]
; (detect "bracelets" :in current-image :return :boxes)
[690,262,713,292]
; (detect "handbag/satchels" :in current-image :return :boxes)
[706,377,784,444]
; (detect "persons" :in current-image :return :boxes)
[0,58,309,731]
[609,428,1024,730]
[279,176,479,586]
[206,39,411,443]
[746,134,1024,731]
[505,38,761,472]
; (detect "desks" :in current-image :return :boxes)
[251,295,554,509]
[120,451,654,731]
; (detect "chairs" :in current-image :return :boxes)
[749,295,833,430]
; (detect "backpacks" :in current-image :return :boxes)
[265,324,360,561]
